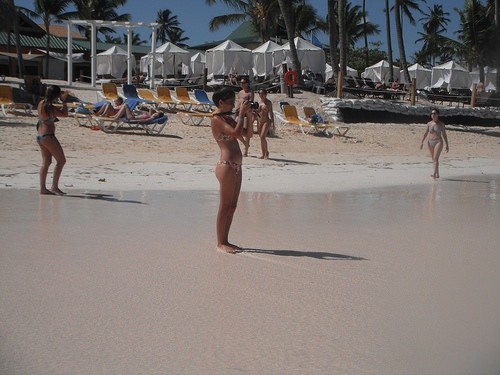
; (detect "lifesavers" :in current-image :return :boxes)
[284,71,298,86]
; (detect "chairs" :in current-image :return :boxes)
[223,71,472,136]
[0,71,217,137]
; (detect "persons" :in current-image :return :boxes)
[375,81,383,90]
[36,85,69,194]
[228,74,238,85]
[287,68,294,98]
[478,80,483,92]
[133,73,147,83]
[420,108,449,179]
[392,79,400,98]
[234,75,275,159]
[211,87,254,253]
[93,96,164,121]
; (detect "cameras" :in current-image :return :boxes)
[242,99,259,110]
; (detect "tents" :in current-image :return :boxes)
[140,37,357,81]
[361,60,497,92]
[96,45,136,78]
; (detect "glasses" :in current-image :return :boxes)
[239,81,248,84]
[431,113,437,115]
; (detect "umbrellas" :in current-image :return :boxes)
[6,11,46,54]
